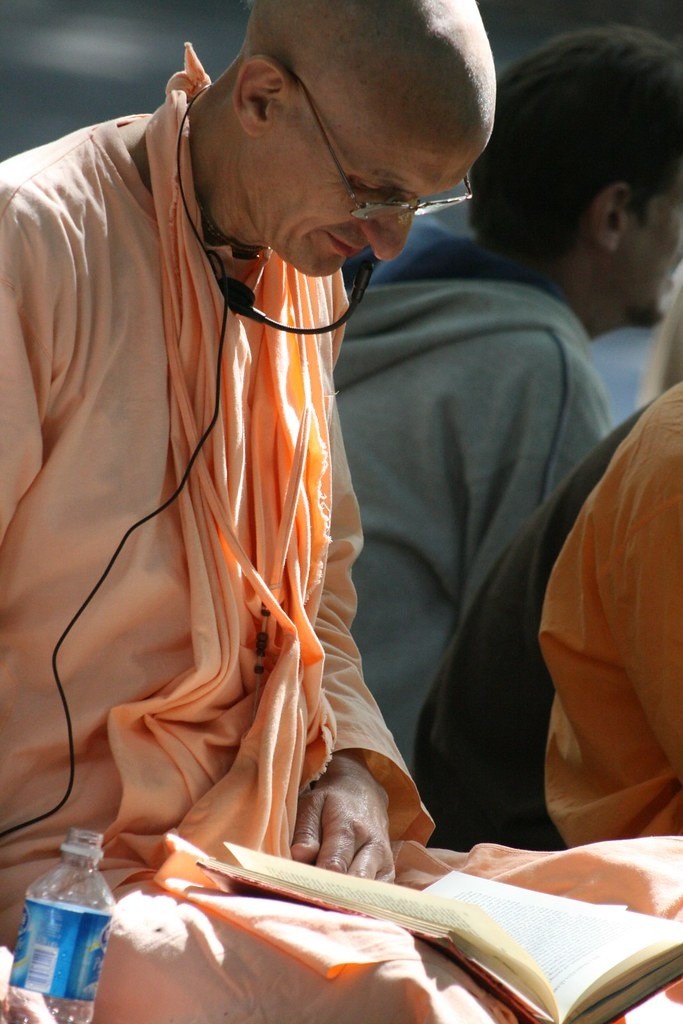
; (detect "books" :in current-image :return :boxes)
[197,839,683,1024]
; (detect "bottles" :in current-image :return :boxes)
[7,828,117,1024]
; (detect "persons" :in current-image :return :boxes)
[0,0,683,1024]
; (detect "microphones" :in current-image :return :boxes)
[219,259,374,334]
